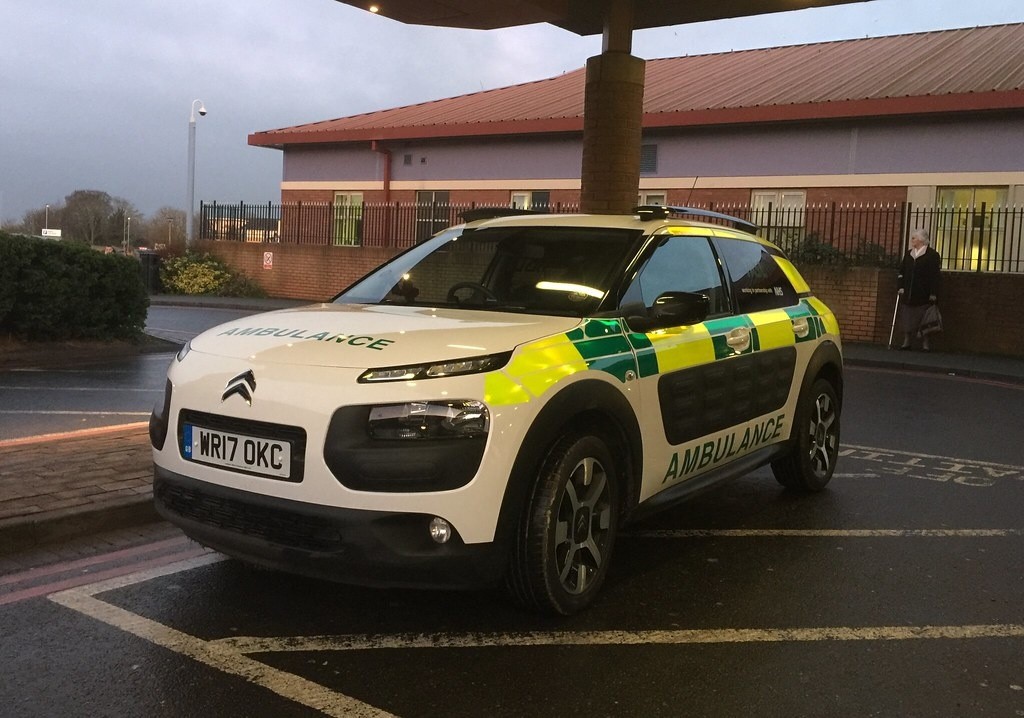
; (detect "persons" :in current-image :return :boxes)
[898,229,941,352]
[454,240,601,308]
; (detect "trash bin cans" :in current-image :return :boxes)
[139,252,161,296]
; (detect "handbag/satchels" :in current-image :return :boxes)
[917,300,945,342]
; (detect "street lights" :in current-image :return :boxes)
[185,98,207,253]
[45,204,50,228]
[127,216,131,251]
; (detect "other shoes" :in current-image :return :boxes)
[896,345,913,351]
[920,347,931,353]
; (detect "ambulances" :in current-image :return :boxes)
[148,205,846,622]
